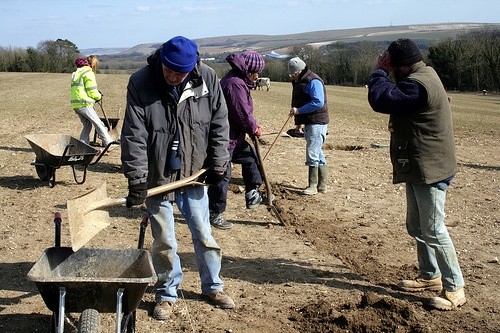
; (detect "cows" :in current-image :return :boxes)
[254,78,271,91]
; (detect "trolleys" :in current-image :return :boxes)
[90,107,121,146]
[24,133,120,188]
[27,211,159,333]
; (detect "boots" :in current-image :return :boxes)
[301,167,319,195]
[318,165,329,194]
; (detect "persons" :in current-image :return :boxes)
[288,57,328,194]
[70,56,119,152]
[121,37,266,320]
[368,38,467,309]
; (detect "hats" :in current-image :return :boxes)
[288,57,306,74]
[239,50,265,73]
[388,37,423,66]
[159,35,199,74]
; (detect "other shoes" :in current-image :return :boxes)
[105,140,120,152]
[428,287,467,310]
[201,290,236,309]
[398,276,443,292]
[209,210,234,229]
[153,300,174,319]
[245,187,276,209]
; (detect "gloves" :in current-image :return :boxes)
[126,194,146,208]
[205,170,227,185]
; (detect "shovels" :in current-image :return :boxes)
[66,168,211,252]
[98,91,120,142]
[252,135,286,227]
